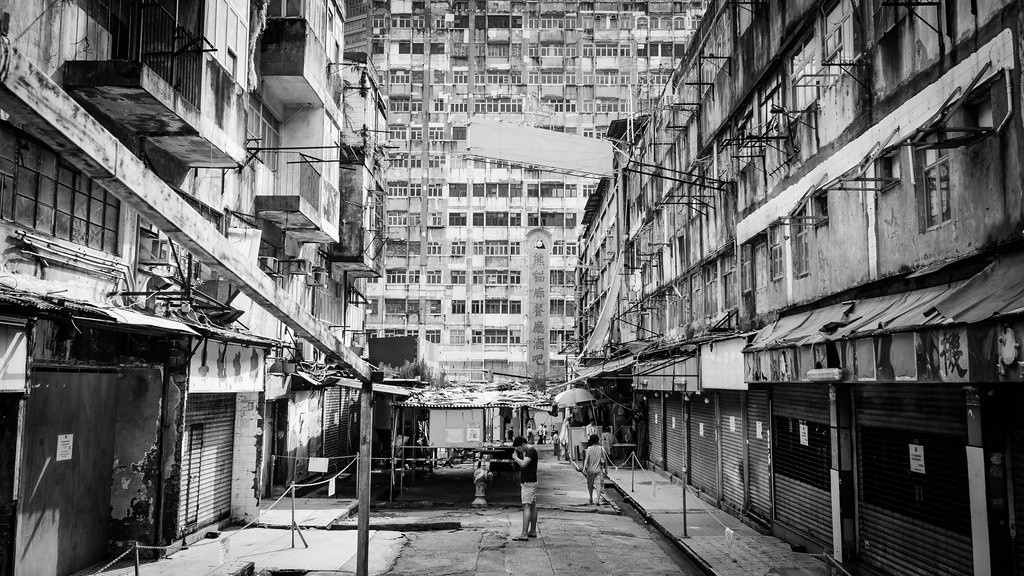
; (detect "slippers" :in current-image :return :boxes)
[512,537,529,541]
[527,532,537,538]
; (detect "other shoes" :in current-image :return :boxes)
[596,501,599,505]
[545,442,546,444]
[540,443,543,444]
[537,443,539,444]
[549,442,551,444]
[589,499,593,503]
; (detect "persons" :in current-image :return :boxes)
[512,436,538,541]
[508,424,559,456]
[582,434,608,505]
[586,418,600,443]
[597,426,617,476]
[623,428,635,461]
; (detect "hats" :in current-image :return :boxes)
[544,425,547,426]
[541,424,543,425]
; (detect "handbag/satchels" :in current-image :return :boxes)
[600,448,606,465]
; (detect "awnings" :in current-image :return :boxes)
[569,357,639,380]
[0,282,287,352]
[743,257,1024,385]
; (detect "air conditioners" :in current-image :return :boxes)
[257,256,279,274]
[662,94,674,110]
[306,271,329,287]
[627,291,636,304]
[289,259,312,276]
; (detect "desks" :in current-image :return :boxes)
[613,444,637,469]
